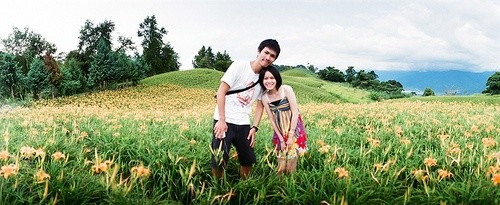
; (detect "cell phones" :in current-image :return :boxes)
[218,131,226,138]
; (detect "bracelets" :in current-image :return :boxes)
[288,128,295,138]
[250,126,258,132]
[279,138,284,142]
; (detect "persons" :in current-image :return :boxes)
[260,67,306,175]
[212,39,280,176]
